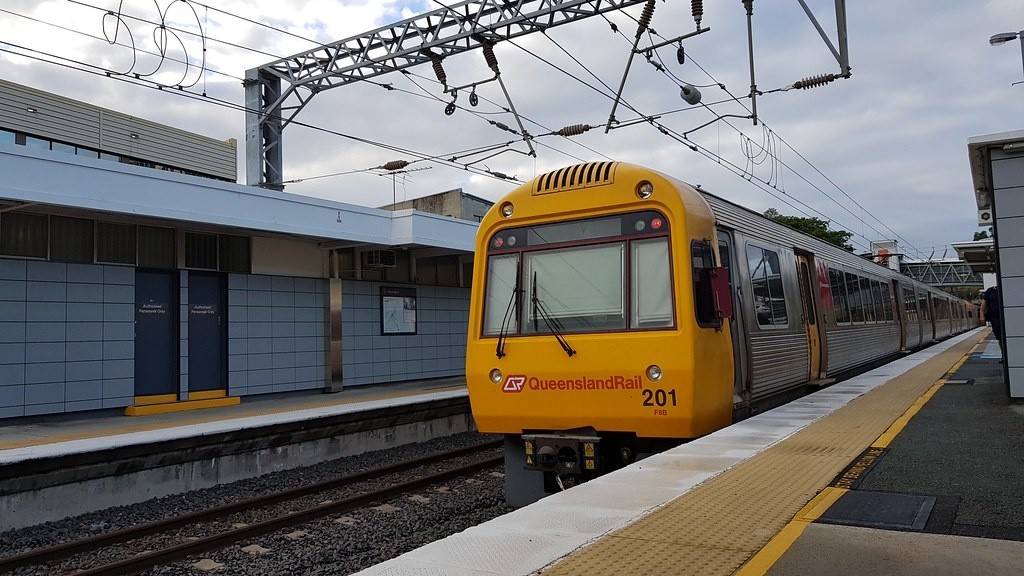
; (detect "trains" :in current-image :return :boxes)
[464,161,986,494]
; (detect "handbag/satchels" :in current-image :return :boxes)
[985,307,991,321]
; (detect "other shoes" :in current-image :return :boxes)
[999,359,1002,363]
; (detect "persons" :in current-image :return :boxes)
[980,286,1004,364]
